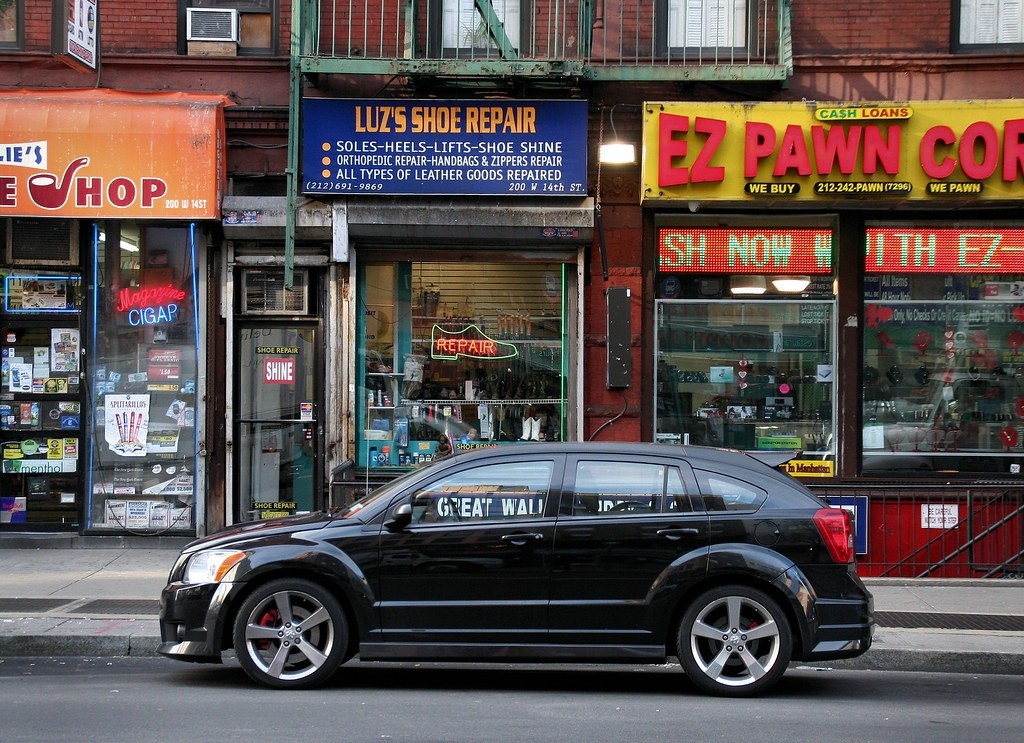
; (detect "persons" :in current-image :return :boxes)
[493,407,512,441]
[729,407,756,419]
[765,407,792,418]
[534,407,554,442]
[432,370,440,381]
[367,363,386,397]
[475,362,487,383]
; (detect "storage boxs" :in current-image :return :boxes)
[10,363,33,394]
[104,500,192,530]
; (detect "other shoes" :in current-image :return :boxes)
[423,389,432,399]
[545,425,555,442]
[440,388,448,400]
[502,418,518,441]
[449,390,457,400]
[431,389,441,399]
[537,380,547,399]
[458,393,465,400]
[527,380,538,399]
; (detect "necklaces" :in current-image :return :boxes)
[918,335,928,346]
[975,336,986,346]
[967,370,980,381]
[919,371,928,383]
[1009,333,1021,344]
[1004,430,1015,442]
[889,368,902,382]
[1020,400,1024,409]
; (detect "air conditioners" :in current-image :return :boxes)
[186,7,241,44]
[241,268,308,316]
[7,217,80,265]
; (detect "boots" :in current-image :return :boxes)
[519,416,532,441]
[530,417,542,442]
[513,375,528,399]
[486,367,500,400]
[503,371,514,400]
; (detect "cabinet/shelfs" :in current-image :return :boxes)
[359,258,570,472]
[91,365,197,530]
[0,310,87,533]
[654,299,1024,490]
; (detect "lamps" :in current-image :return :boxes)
[731,276,766,295]
[772,275,812,292]
[99,231,139,252]
[598,104,636,166]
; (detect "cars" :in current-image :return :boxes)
[158,440,877,698]
